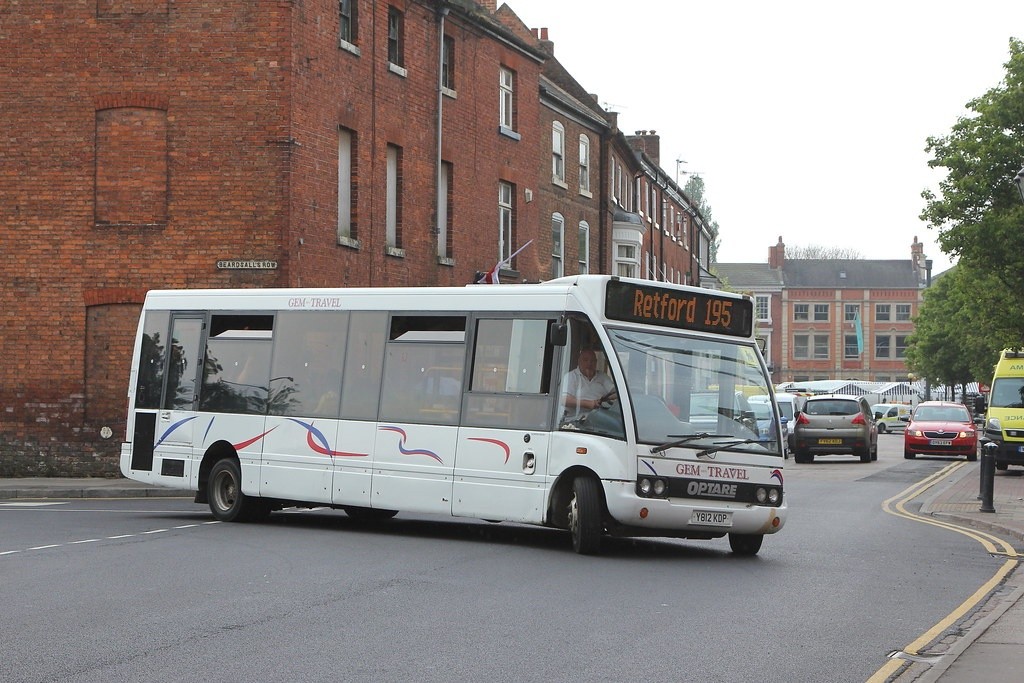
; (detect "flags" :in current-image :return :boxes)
[854,313,864,354]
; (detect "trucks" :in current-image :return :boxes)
[982,347,1024,471]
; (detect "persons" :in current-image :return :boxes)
[556,350,616,429]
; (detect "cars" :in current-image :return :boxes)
[678,388,796,455]
[867,401,917,434]
[903,399,978,463]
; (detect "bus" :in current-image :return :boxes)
[119,273,788,555]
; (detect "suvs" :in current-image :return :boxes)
[793,393,882,464]
